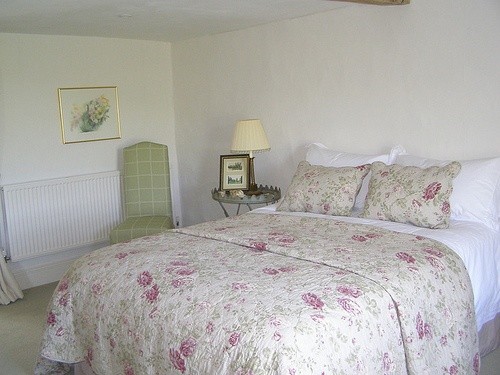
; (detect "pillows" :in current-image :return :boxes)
[275,161,369,217]
[358,161,461,230]
[305,141,403,211]
[389,145,499,235]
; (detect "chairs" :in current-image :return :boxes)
[111,140,174,245]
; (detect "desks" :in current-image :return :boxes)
[211,184,282,218]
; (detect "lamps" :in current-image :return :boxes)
[230,119,272,195]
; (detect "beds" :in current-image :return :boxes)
[25,137,500,375]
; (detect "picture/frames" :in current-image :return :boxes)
[220,154,250,191]
[57,84,123,144]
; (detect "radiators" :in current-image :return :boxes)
[2,170,122,264]
